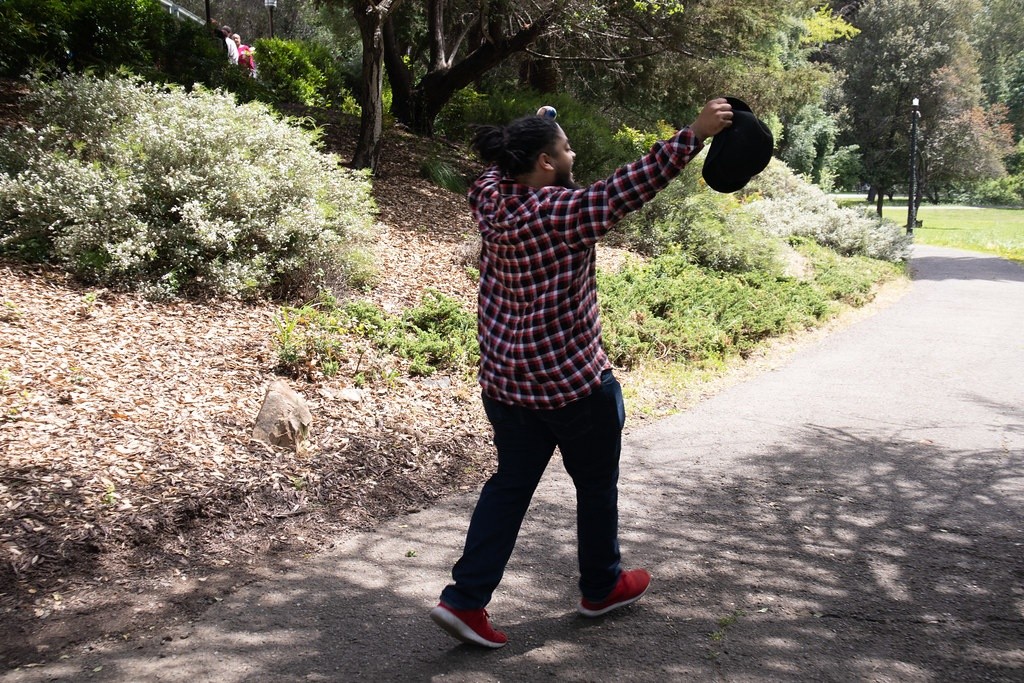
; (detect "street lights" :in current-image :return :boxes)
[906,98,919,235]
[264,0,278,39]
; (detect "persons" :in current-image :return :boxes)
[430,98,733,648]
[210,18,257,78]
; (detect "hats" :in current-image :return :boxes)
[703,97,774,193]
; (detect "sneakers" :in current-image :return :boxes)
[579,568,651,616]
[430,601,508,648]
